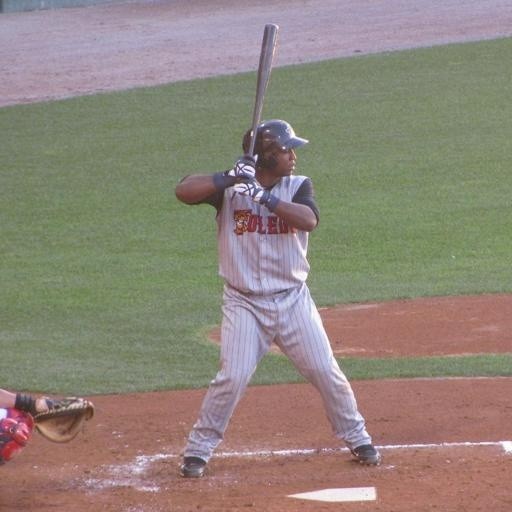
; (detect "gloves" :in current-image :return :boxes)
[213,155,258,190]
[234,179,281,214]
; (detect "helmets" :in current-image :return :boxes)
[241,119,310,163]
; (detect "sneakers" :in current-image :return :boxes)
[179,456,208,478]
[350,442,382,467]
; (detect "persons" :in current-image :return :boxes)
[174,120,383,477]
[0,387,62,467]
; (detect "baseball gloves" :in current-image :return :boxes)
[30,396,95,443]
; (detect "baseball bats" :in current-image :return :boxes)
[248,23,280,156]
